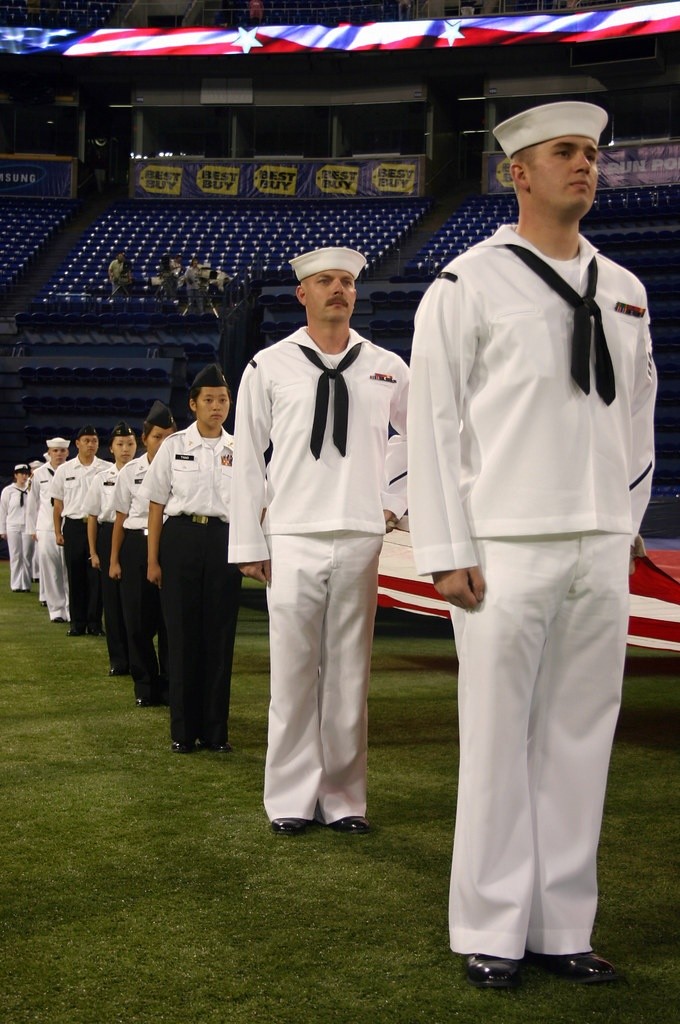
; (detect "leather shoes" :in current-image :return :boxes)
[465,951,522,989]
[9,571,232,755]
[315,813,372,834]
[271,816,309,835]
[523,951,619,984]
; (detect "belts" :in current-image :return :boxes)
[79,519,88,524]
[176,512,228,527]
[125,528,143,536]
[101,521,115,528]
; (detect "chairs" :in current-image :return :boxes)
[0,184,680,496]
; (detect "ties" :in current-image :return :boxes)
[14,487,29,507]
[506,243,617,407]
[47,468,55,506]
[297,342,367,461]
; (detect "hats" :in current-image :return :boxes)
[492,101,610,158]
[43,452,51,461]
[191,363,228,387]
[46,437,71,448]
[111,420,135,436]
[29,460,44,468]
[15,464,28,470]
[146,401,175,429]
[76,424,97,441]
[289,246,368,280]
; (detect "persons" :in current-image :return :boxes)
[0,437,72,624]
[79,421,137,676]
[109,253,131,297]
[226,247,411,834]
[48,426,115,636]
[109,400,174,708]
[169,254,224,315]
[405,102,660,994]
[140,364,267,753]
